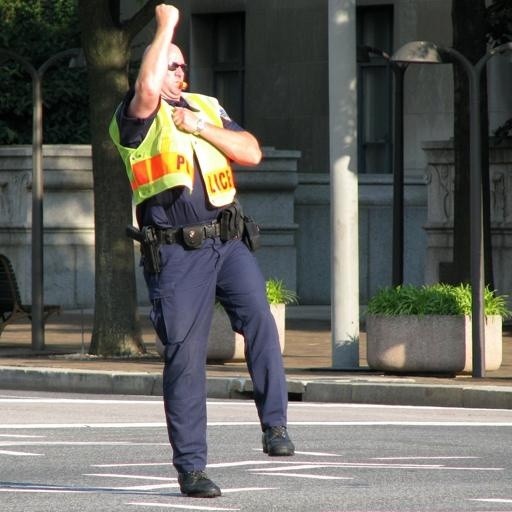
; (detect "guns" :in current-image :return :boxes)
[126,222,160,275]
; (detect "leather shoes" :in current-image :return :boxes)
[176,469,222,499]
[261,425,296,458]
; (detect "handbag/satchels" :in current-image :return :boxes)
[241,214,263,253]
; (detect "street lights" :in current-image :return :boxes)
[31,47,91,353]
[389,38,512,377]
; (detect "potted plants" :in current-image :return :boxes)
[153,273,304,363]
[360,275,512,375]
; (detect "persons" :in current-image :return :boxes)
[105,2,296,500]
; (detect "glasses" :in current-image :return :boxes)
[166,61,189,73]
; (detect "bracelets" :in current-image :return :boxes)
[192,117,205,136]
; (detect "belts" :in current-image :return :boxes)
[149,221,222,246]
